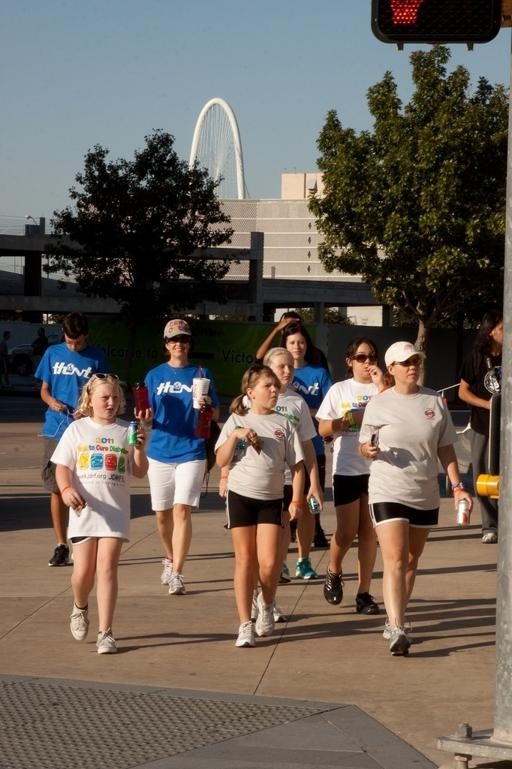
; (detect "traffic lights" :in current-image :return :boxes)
[370,0,504,44]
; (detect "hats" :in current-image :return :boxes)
[164,318,191,340]
[384,340,427,366]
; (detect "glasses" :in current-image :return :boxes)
[401,358,422,367]
[167,336,190,344]
[91,372,120,384]
[351,355,378,363]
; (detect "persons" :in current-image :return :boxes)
[457,312,503,545]
[314,337,389,616]
[49,373,149,655]
[134,319,219,594]
[59,484,71,495]
[214,312,332,648]
[0,330,12,388]
[356,342,473,657]
[35,311,109,566]
[32,326,48,386]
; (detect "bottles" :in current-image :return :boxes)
[135,380,150,418]
[307,495,321,514]
[197,405,211,439]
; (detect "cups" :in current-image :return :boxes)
[192,377,211,408]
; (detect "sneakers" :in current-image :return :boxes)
[161,558,174,586]
[295,557,318,580]
[69,601,89,641]
[323,565,344,604]
[278,562,291,583]
[313,532,329,548]
[97,630,117,655]
[388,628,410,656]
[234,590,286,648]
[47,544,70,565]
[354,592,380,615]
[167,571,186,595]
[481,531,498,544]
[382,621,412,647]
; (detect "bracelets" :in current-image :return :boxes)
[360,442,367,459]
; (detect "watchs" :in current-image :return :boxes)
[452,482,467,491]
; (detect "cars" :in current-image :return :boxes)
[7,343,41,376]
